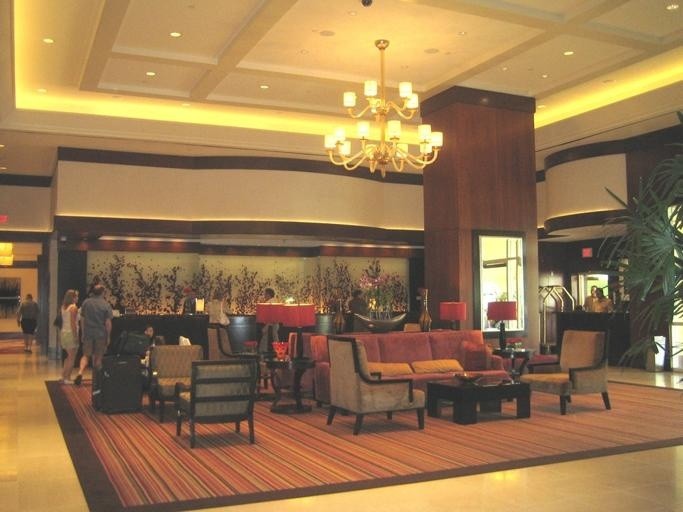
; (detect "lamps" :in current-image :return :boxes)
[0,242,12,256]
[325,40,444,179]
[282,304,316,360]
[488,302,517,349]
[440,302,466,330]
[0,254,13,266]
[256,304,282,357]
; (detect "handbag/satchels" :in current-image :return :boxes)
[106,331,151,359]
[53,315,62,328]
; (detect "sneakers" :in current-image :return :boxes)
[24,349,32,354]
[58,375,82,385]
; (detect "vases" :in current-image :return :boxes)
[354,314,407,333]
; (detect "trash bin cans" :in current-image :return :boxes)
[541,343,559,356]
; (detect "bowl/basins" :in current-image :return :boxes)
[454,374,483,385]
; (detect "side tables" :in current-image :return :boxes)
[266,361,316,414]
[493,349,536,382]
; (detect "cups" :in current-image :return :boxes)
[273,341,288,361]
[512,370,520,384]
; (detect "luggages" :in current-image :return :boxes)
[92,354,142,414]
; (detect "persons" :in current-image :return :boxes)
[16,294,40,352]
[59,289,80,384]
[140,326,165,358]
[182,291,197,314]
[592,288,614,312]
[73,284,114,386]
[258,287,279,353]
[584,286,598,311]
[346,290,368,331]
[205,289,234,327]
[115,296,126,316]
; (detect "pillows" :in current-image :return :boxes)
[364,363,412,375]
[411,359,464,374]
[462,340,490,371]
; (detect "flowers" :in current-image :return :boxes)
[352,272,408,319]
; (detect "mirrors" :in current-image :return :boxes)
[471,229,528,339]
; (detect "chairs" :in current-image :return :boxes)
[174,360,256,448]
[520,328,611,415]
[150,345,204,423]
[327,335,426,435]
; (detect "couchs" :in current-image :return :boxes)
[310,330,502,415]
[276,332,321,398]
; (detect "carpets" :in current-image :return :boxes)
[46,381,683,512]
